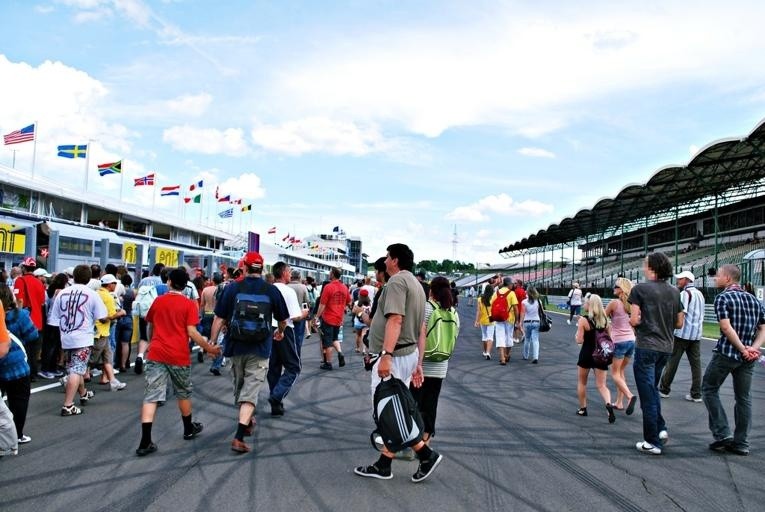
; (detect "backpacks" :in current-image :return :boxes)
[423,300,458,362]
[585,315,614,365]
[228,280,272,343]
[131,286,157,318]
[491,290,513,322]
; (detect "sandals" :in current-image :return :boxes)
[576,408,587,416]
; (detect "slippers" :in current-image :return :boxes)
[136,443,157,455]
[183,423,203,440]
[605,396,637,423]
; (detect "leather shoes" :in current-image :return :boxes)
[245,416,257,436]
[232,438,251,452]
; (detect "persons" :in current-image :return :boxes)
[347,244,460,482]
[702,264,765,456]
[1,252,351,453]
[467,277,543,366]
[566,253,706,455]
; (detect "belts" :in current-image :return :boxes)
[524,320,539,322]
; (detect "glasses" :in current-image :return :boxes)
[614,284,620,288]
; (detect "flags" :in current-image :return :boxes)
[267,226,276,234]
[183,194,201,204]
[282,235,300,243]
[160,185,180,196]
[189,180,203,192]
[57,144,87,158]
[134,174,154,186]
[3,124,34,146]
[98,161,121,176]
[215,187,251,218]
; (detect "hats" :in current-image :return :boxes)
[244,252,263,269]
[101,274,119,284]
[572,282,580,288]
[64,267,74,276]
[360,290,368,296]
[33,269,52,278]
[674,271,695,282]
[21,258,36,267]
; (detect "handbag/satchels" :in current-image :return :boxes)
[540,314,553,331]
[372,375,423,452]
[566,301,571,305]
[364,352,380,371]
[489,316,494,322]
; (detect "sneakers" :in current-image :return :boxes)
[305,333,311,339]
[19,435,31,443]
[79,390,95,403]
[636,430,668,455]
[567,319,579,327]
[354,465,393,480]
[134,357,143,375]
[710,438,749,456]
[198,351,204,362]
[61,404,83,415]
[395,449,442,482]
[268,395,285,418]
[209,368,220,375]
[482,351,510,365]
[513,335,525,343]
[320,352,344,370]
[38,361,127,391]
[531,359,538,363]
[686,395,702,403]
[657,390,671,398]
